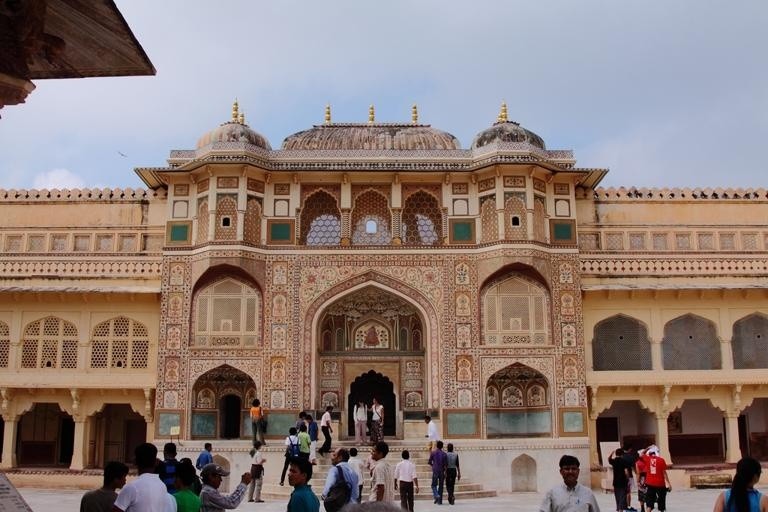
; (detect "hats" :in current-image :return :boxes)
[200,462,230,476]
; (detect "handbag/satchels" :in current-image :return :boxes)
[256,420,267,433]
[250,464,264,479]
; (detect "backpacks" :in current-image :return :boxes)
[288,437,300,457]
[323,465,353,512]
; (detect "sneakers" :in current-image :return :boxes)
[279,483,283,486]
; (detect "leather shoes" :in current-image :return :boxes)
[255,500,263,503]
[248,500,254,502]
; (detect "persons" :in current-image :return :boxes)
[711,454,768,512]
[609,443,674,512]
[79,443,248,511]
[248,396,461,512]
[538,454,601,511]
